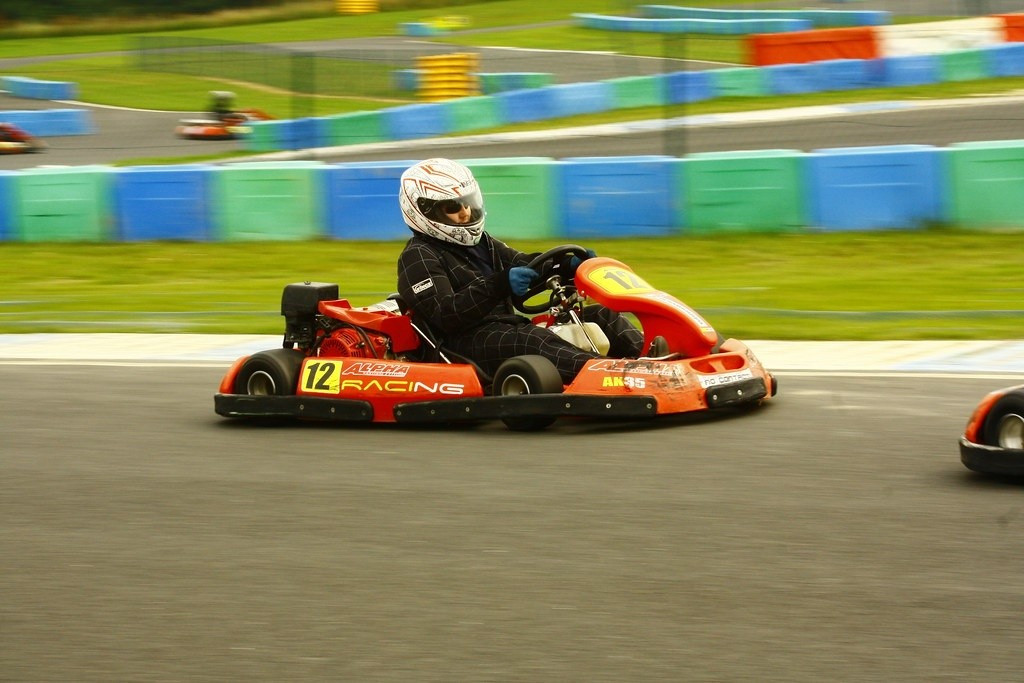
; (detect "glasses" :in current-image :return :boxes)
[439,200,470,214]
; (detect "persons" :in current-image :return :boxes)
[206,91,237,120]
[396,156,672,386]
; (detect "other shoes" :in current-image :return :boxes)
[644,336,669,358]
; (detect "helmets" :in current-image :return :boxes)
[399,158,486,246]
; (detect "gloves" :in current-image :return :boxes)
[570,249,596,271]
[508,265,539,296]
